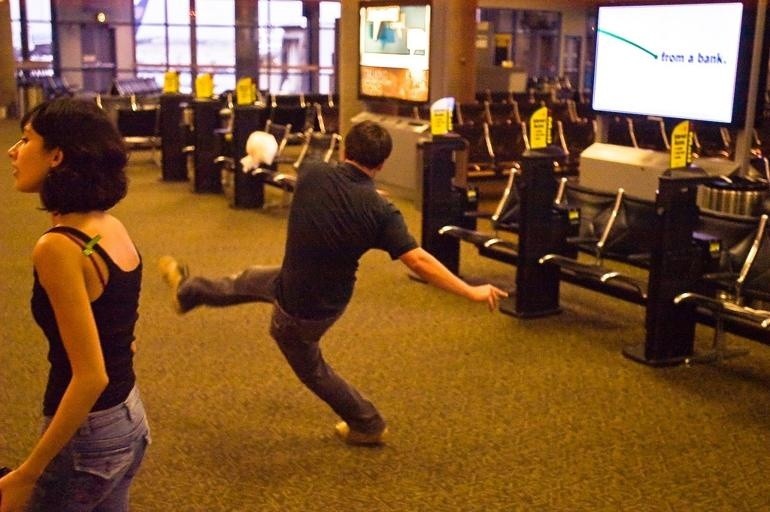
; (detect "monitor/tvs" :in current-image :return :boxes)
[356,1,432,104]
[588,2,756,130]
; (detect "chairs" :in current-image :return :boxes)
[92,90,342,210]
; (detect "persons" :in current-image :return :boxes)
[0,95,153,511]
[160,120,510,444]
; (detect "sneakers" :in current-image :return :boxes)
[158,255,193,314]
[335,421,389,445]
